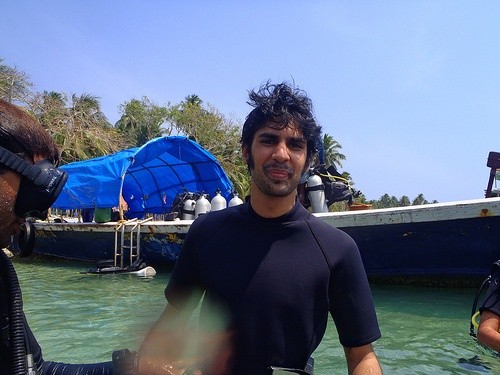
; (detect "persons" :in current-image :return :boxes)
[478,260,500,351]
[0,98,229,375]
[146,75,386,375]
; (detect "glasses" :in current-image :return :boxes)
[14,160,69,221]
